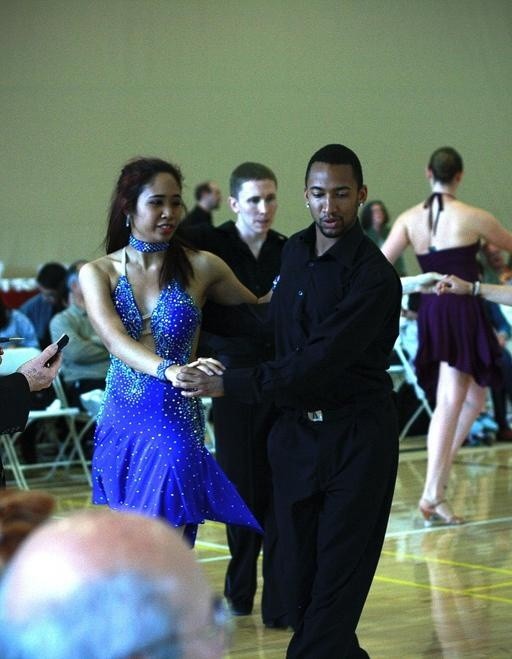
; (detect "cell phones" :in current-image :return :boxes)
[41,332,69,367]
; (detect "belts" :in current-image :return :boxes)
[297,408,345,424]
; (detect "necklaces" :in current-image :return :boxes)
[129,236,169,254]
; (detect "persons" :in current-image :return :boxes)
[174,142,401,659]
[1,509,227,659]
[362,145,512,526]
[1,259,112,559]
[78,152,275,546]
[198,163,292,631]
[179,179,221,251]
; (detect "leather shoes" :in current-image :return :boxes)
[264,615,284,627]
[231,600,253,615]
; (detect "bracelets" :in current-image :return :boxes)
[157,359,176,382]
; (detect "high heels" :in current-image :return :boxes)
[420,497,464,525]
